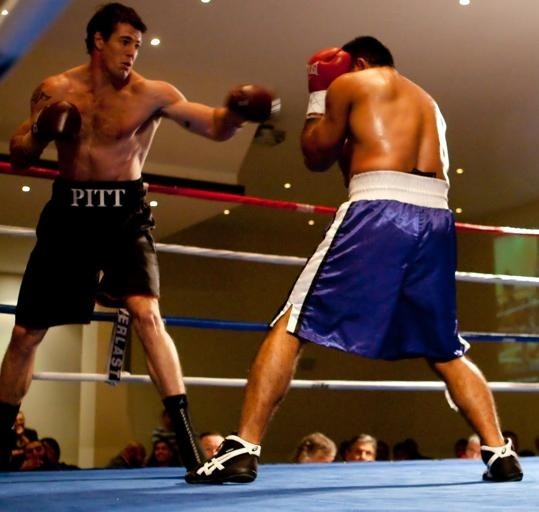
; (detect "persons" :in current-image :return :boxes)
[184,35,525,486]
[199,430,225,458]
[110,428,179,469]
[0,0,277,485]
[10,412,79,470]
[294,432,432,464]
[456,431,535,458]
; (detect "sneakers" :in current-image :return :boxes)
[480,436,522,481]
[184,435,262,484]
[169,409,207,471]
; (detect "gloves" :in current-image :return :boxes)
[306,47,352,92]
[31,100,81,145]
[337,136,353,187]
[225,83,270,122]
